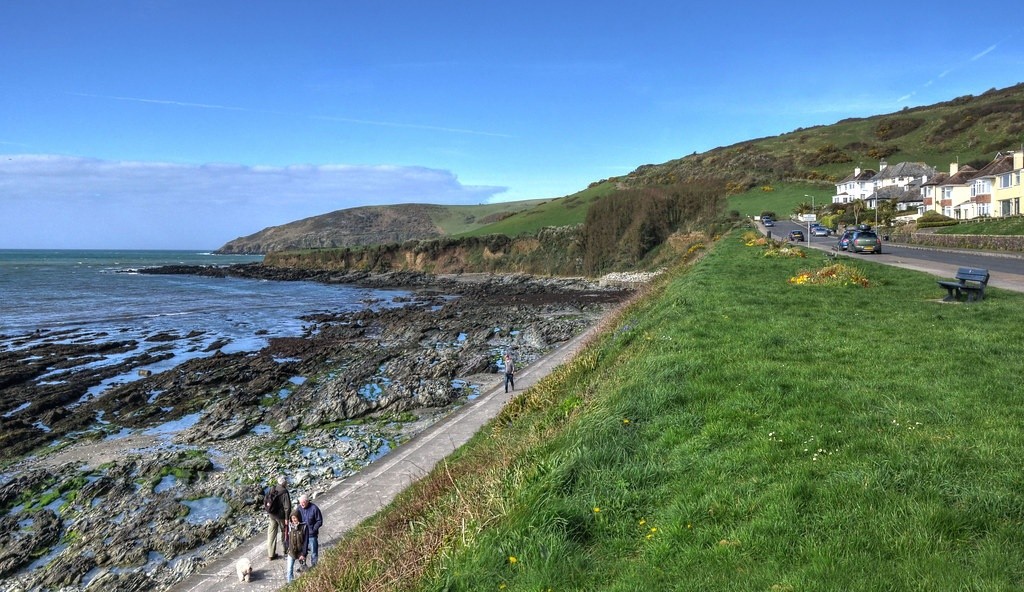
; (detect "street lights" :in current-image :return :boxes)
[804,194,814,214]
[862,189,878,236]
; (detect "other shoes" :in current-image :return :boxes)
[296,568,301,573]
[282,551,287,557]
[269,554,278,561]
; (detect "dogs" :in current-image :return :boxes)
[236,558,252,583]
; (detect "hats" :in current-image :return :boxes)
[297,495,309,501]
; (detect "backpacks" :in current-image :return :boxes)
[264,486,288,514]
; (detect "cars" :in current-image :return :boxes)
[789,230,804,241]
[838,225,881,254]
[810,224,836,237]
[761,216,773,227]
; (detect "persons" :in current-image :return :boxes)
[265,477,291,559]
[286,510,309,582]
[292,495,323,566]
[504,355,514,393]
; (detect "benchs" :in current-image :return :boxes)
[937,266,990,302]
